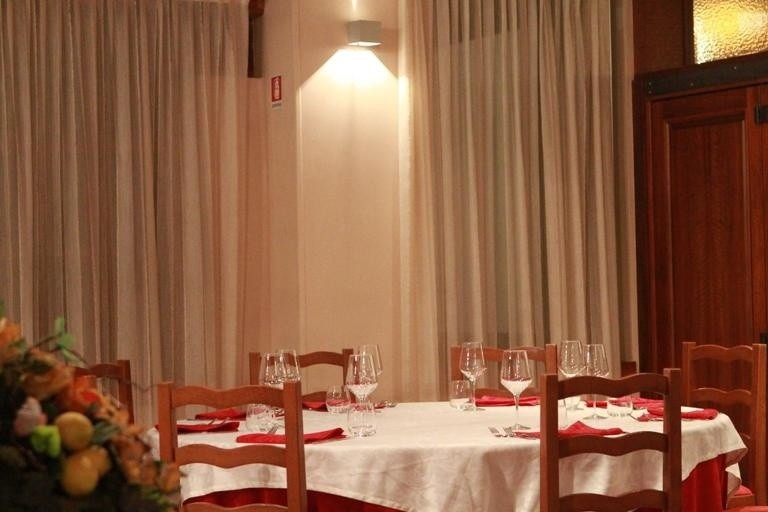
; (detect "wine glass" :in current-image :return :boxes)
[445,338,634,443]
[244,343,397,445]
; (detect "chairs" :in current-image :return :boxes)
[244,346,357,406]
[69,355,134,432]
[155,377,310,512]
[446,343,557,399]
[684,336,767,512]
[534,366,684,512]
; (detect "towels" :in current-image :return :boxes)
[194,404,266,419]
[236,428,350,444]
[152,419,241,435]
[583,393,666,408]
[301,398,387,412]
[514,420,632,440]
[636,402,718,423]
[475,394,541,406]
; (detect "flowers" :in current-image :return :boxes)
[1,299,189,512]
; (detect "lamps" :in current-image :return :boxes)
[346,20,384,47]
